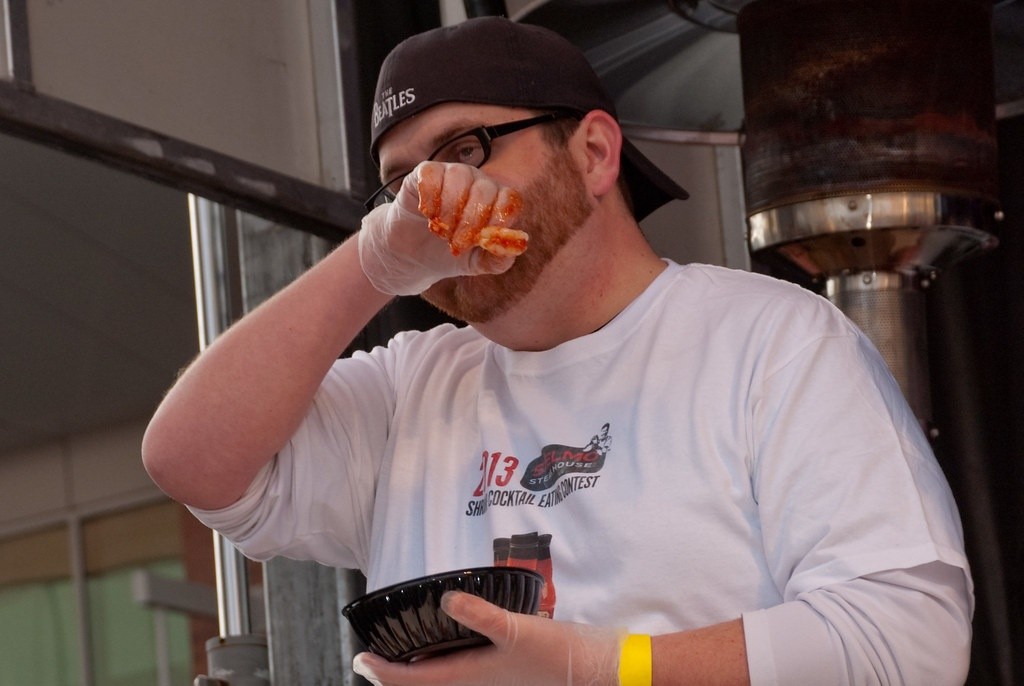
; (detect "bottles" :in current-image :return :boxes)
[493,531,556,618]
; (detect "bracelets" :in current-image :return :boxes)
[618,634,652,686]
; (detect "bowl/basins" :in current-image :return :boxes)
[341,566,544,661]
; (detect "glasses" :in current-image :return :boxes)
[365,110,582,214]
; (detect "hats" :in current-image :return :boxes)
[369,18,691,227]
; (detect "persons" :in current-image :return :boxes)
[143,20,974,686]
